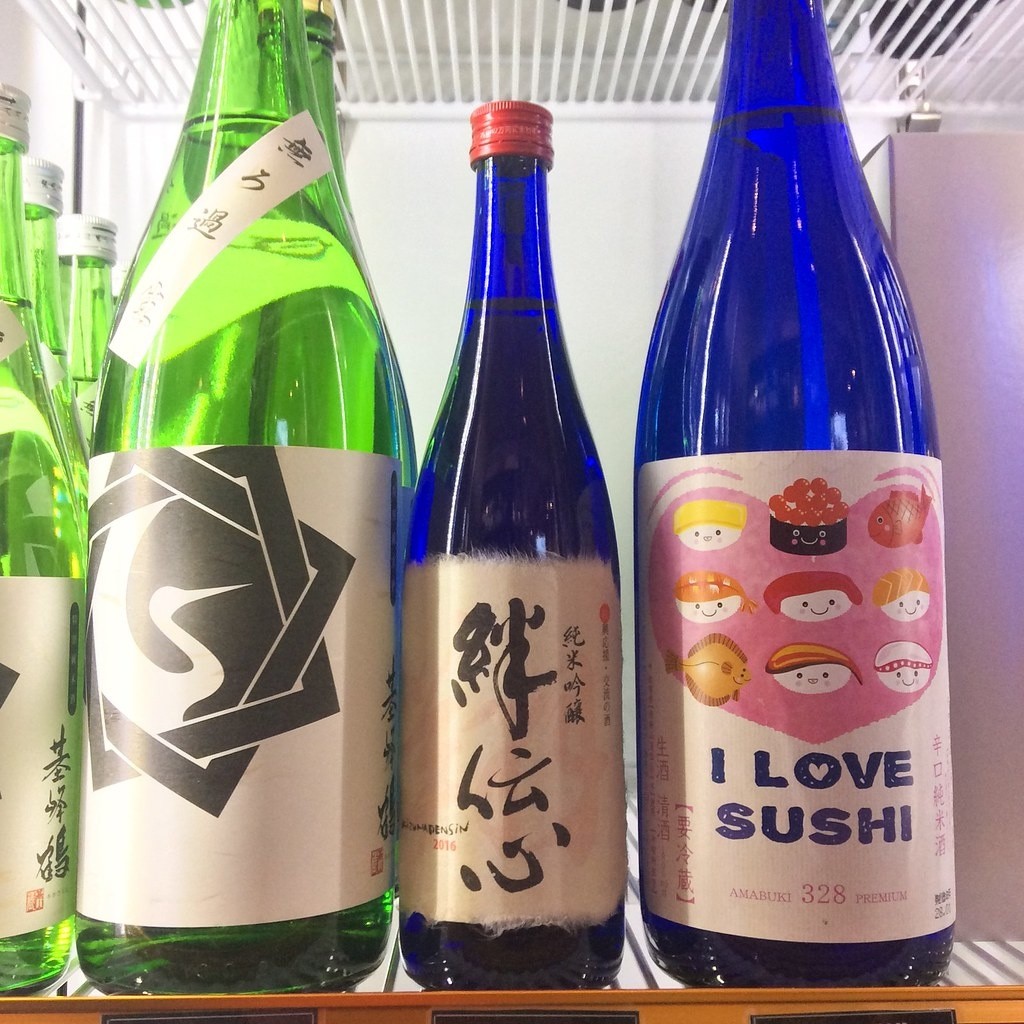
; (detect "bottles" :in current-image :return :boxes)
[0,0,416,997]
[391,99,630,990]
[626,0,957,987]
